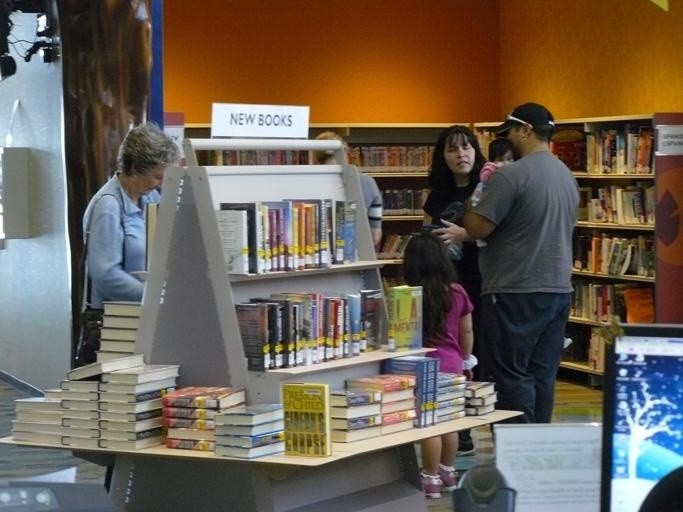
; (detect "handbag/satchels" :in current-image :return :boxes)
[74,308,103,368]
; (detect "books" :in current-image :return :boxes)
[352,129,497,289]
[282,356,498,458]
[233,288,381,370]
[549,127,656,370]
[204,150,301,166]
[11,301,285,459]
[218,197,356,275]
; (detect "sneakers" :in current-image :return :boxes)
[420,466,457,499]
[456,436,476,457]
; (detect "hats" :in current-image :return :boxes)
[495,102,555,137]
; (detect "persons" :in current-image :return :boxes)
[460,100,583,425]
[422,125,516,378]
[315,133,381,245]
[80,121,181,365]
[400,234,474,502]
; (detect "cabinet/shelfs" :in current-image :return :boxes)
[1,137,525,512]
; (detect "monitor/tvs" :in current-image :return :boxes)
[599,322,682,512]
